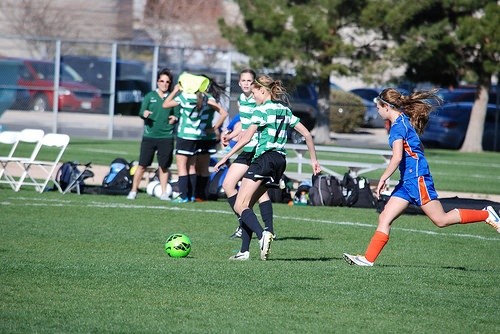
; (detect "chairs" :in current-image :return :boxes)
[0,128,71,194]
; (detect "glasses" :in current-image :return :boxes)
[158,79,171,83]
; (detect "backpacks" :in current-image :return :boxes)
[98,158,136,195]
[47,160,95,195]
[308,170,348,208]
[339,169,379,208]
[202,156,313,207]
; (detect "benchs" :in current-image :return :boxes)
[147,138,396,193]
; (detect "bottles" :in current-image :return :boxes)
[358,176,364,189]
[300,191,307,203]
[286,180,299,202]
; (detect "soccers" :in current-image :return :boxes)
[145,180,161,196]
[154,182,172,199]
[164,233,192,258]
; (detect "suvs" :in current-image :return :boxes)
[57,53,321,143]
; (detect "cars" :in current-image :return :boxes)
[316,82,500,149]
[1,55,106,113]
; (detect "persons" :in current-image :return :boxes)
[163,72,209,203]
[195,74,228,203]
[214,74,321,261]
[342,88,500,266]
[221,70,278,239]
[126,68,184,200]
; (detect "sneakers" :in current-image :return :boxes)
[482,205,500,234]
[258,226,273,261]
[159,196,172,201]
[342,253,374,267]
[229,226,243,239]
[172,193,197,204]
[126,190,137,200]
[228,250,250,262]
